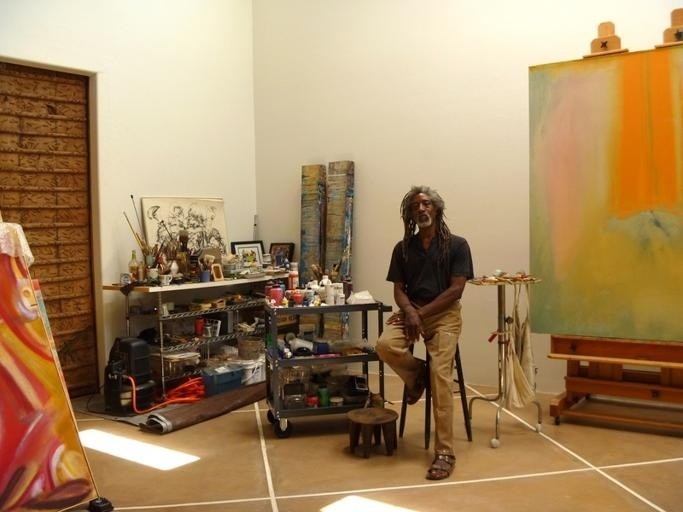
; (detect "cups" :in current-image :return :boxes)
[159,275,173,286]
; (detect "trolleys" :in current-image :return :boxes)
[264,288,393,438]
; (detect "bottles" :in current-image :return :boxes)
[320,275,332,286]
[195,317,205,335]
[289,262,299,289]
[306,383,329,407]
[129,249,138,281]
[202,324,213,337]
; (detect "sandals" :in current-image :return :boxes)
[428,454,455,480]
[408,367,426,404]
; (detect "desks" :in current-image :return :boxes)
[468,278,544,448]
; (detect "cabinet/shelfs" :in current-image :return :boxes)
[103,273,290,403]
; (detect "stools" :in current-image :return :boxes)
[348,408,398,459]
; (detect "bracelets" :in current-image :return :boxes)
[397,301,414,311]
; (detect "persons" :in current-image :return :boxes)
[373,186,472,481]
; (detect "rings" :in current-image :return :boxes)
[396,317,401,322]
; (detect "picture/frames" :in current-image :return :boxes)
[270,243,294,262]
[211,264,224,282]
[232,241,267,269]
